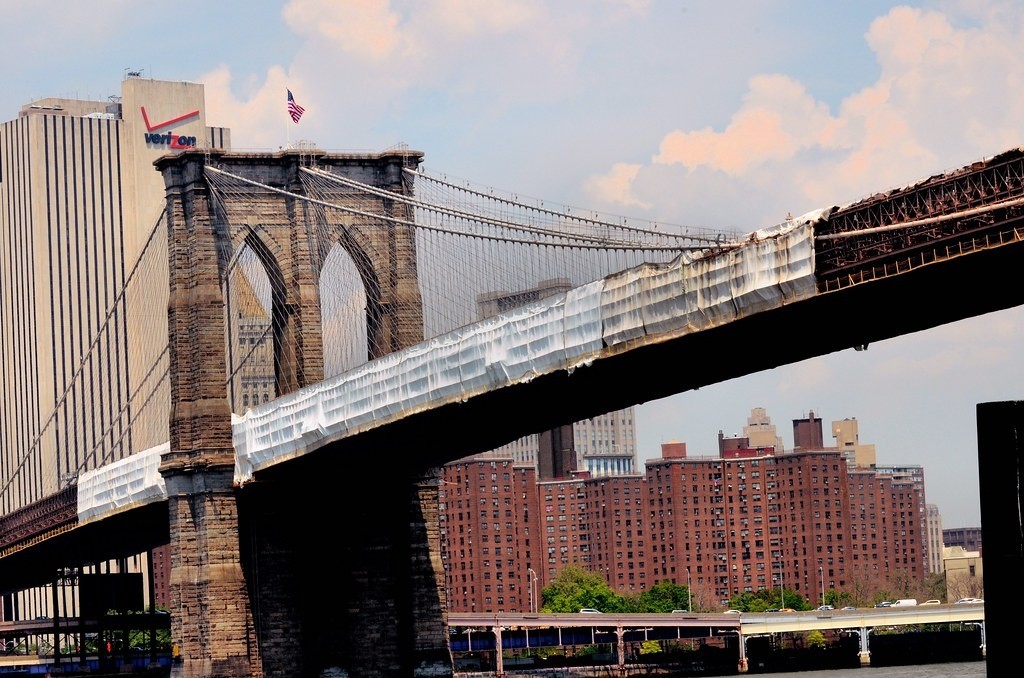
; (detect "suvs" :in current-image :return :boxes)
[919,600,941,605]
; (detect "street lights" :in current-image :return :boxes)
[686,569,692,613]
[528,568,537,613]
[820,566,825,611]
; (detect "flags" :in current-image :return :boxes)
[287,90,305,123]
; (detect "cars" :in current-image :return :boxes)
[765,608,797,612]
[818,605,856,610]
[955,598,984,604]
[724,610,744,615]
[873,602,891,608]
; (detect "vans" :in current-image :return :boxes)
[580,608,613,614]
[672,610,687,613]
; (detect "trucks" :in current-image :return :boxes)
[889,599,917,606]
